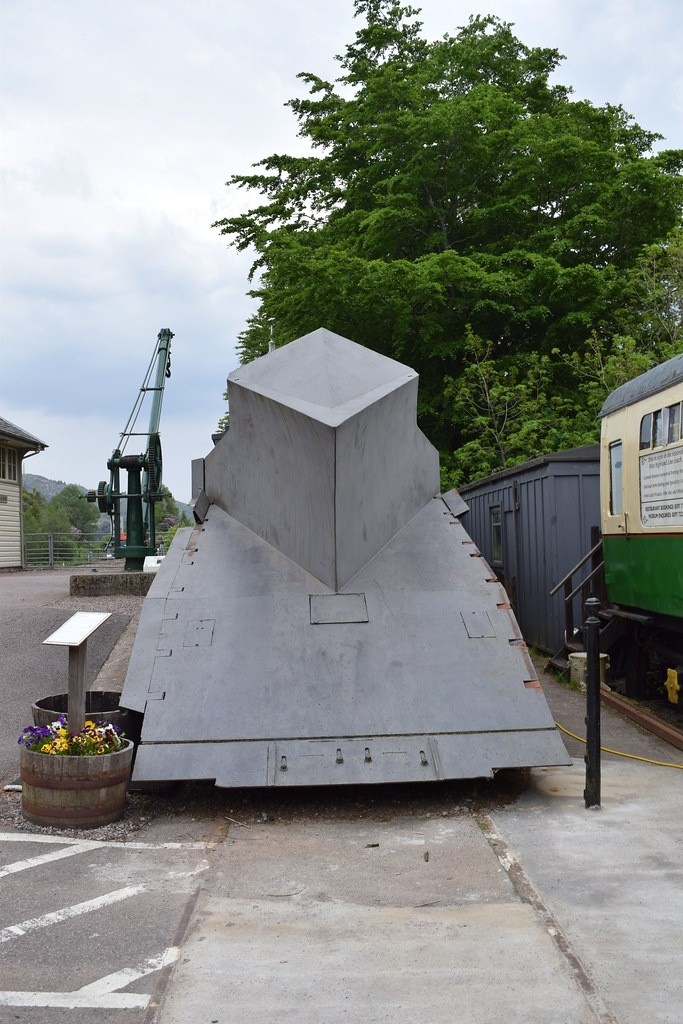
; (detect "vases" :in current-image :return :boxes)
[20,738,136,829]
[34,690,144,771]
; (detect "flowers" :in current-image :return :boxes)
[16,713,127,756]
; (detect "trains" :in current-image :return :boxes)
[596,353,682,715]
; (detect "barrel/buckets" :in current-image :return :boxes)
[29,691,140,737]
[568,652,611,688]
